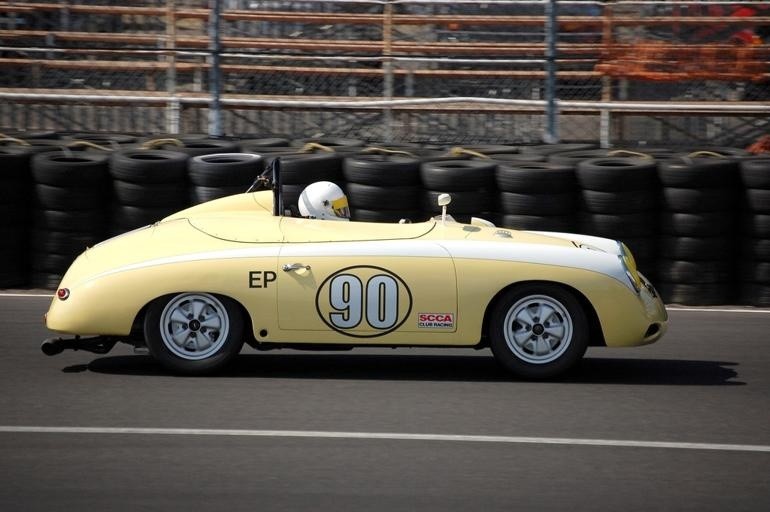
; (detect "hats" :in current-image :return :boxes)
[41,157,668,382]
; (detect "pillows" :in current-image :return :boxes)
[297,180,352,221]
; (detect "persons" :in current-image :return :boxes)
[297,179,412,223]
